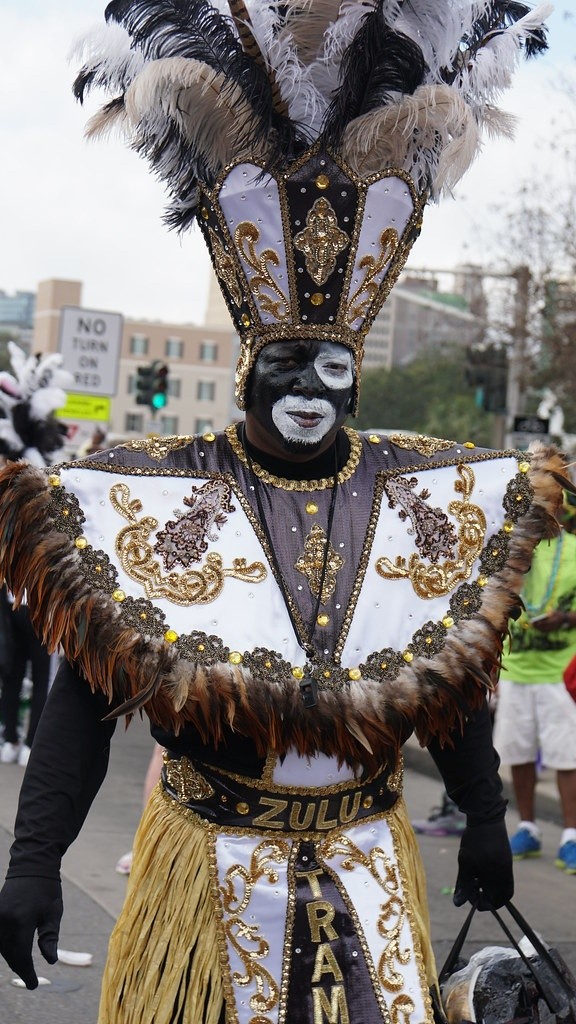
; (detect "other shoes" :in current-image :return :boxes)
[17,746,31,766]
[0,743,17,763]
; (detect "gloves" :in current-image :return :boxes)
[0,875,63,990]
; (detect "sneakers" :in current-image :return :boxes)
[556,841,576,874]
[411,793,466,835]
[510,828,542,861]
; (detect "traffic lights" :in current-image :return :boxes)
[136,366,151,407]
[151,362,169,409]
[464,342,507,411]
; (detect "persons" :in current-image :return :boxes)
[0,330,570,1024]
[405,435,576,878]
[0,339,108,765]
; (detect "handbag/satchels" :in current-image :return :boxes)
[440,901,576,1024]
[452,799,515,911]
